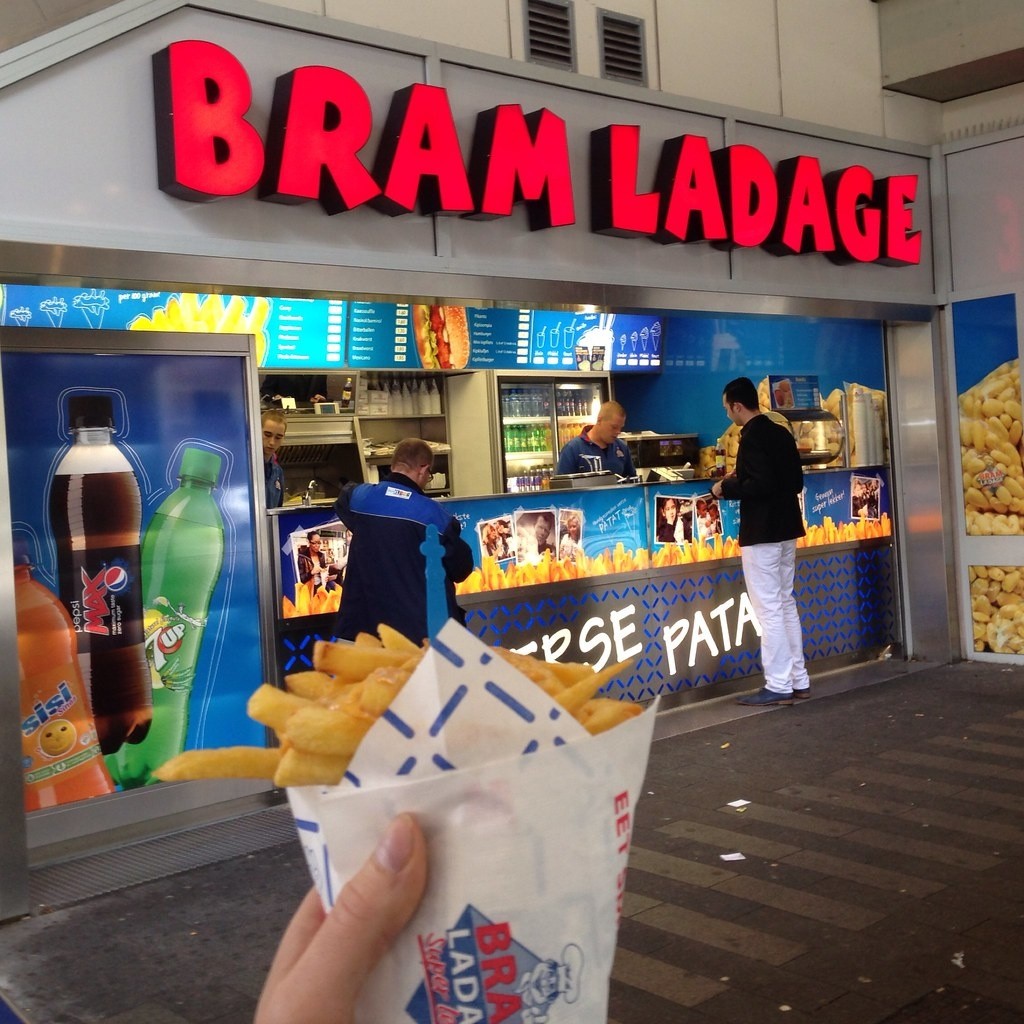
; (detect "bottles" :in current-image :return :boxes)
[100,448,228,783]
[370,464,379,484]
[715,438,727,475]
[50,395,154,753]
[500,425,589,452]
[505,464,555,491]
[16,567,116,813]
[503,389,591,416]
[341,377,352,408]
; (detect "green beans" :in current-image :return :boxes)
[958,359,1024,536]
[968,566,1024,655]
[698,381,889,467]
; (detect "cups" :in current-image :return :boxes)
[853,386,883,466]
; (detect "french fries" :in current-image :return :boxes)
[154,621,647,789]
[797,512,892,546]
[282,582,342,618]
[456,535,741,593]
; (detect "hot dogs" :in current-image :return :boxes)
[413,303,470,369]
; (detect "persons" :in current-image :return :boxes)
[332,438,474,648]
[852,478,878,519]
[482,513,581,563]
[250,809,429,1024]
[657,498,722,543]
[711,377,812,705]
[556,401,637,476]
[289,528,353,586]
[261,410,287,509]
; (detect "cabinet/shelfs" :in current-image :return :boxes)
[356,368,453,498]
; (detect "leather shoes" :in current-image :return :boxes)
[735,688,794,706]
[794,688,810,698]
